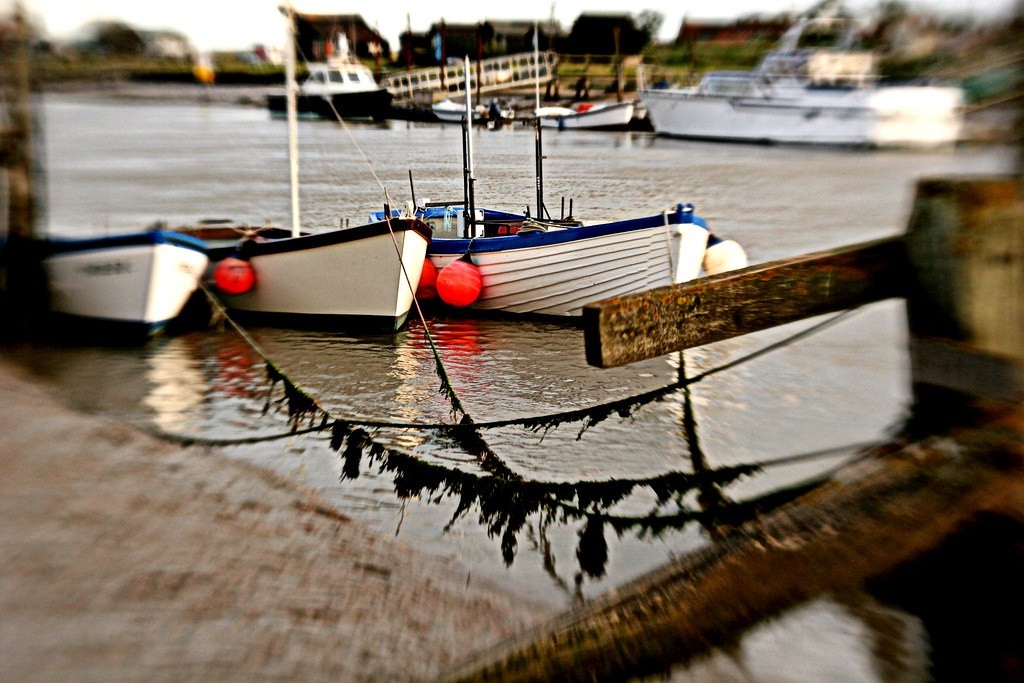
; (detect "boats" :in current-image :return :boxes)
[143,0,436,337]
[264,28,392,125]
[43,223,212,349]
[636,16,967,153]
[429,98,516,129]
[369,16,712,320]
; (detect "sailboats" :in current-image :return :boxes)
[532,25,635,130]
